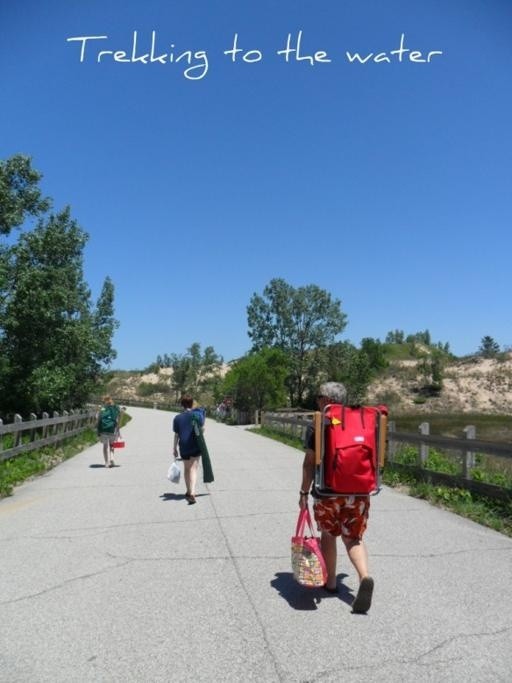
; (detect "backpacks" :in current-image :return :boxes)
[99,405,118,431]
[328,404,376,494]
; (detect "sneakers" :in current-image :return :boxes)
[183,493,196,503]
[325,584,340,593]
[352,577,374,613]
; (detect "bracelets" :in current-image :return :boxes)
[300,491,310,496]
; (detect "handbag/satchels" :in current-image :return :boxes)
[292,508,327,588]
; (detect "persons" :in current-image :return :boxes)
[298,382,374,614]
[97,396,118,468]
[172,392,207,504]
